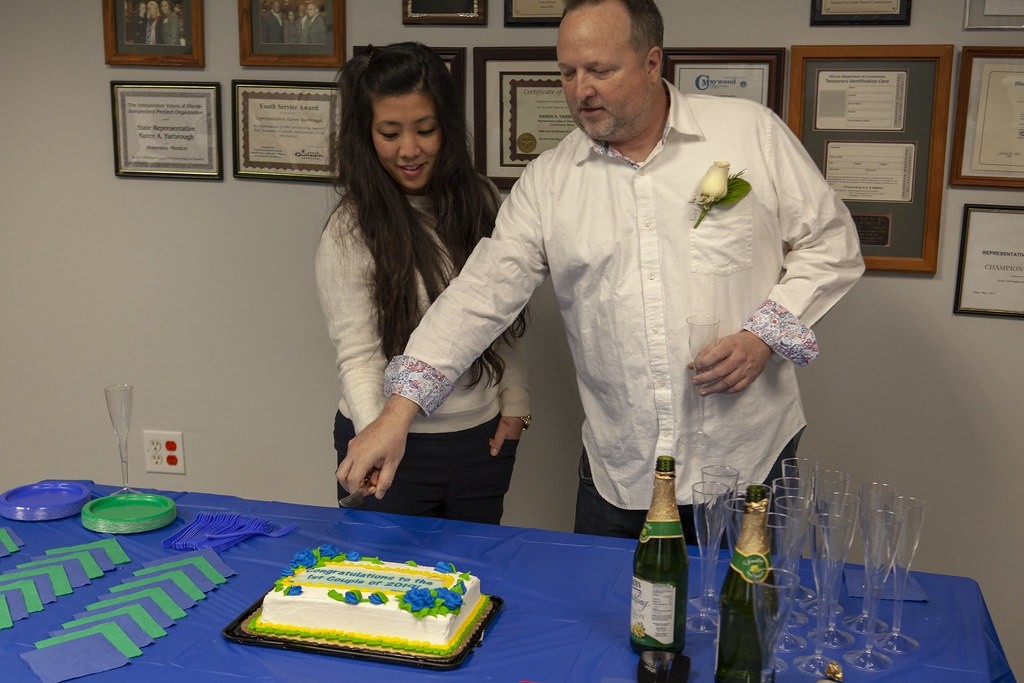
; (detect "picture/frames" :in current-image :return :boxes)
[236,0,348,69]
[102,0,205,69]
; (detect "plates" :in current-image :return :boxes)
[1,483,92,521]
[81,494,178,534]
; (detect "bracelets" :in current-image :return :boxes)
[521,416,531,430]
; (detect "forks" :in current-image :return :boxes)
[161,511,299,555]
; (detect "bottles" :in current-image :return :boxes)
[630,455,687,656]
[714,485,779,683]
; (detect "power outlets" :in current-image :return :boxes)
[143,429,186,476]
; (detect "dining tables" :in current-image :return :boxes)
[0,480,1017,683]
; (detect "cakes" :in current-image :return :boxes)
[248,544,493,657]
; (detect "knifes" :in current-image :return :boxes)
[338,476,373,508]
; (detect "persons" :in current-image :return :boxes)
[128,0,181,46]
[336,1,866,558]
[315,43,528,524]
[261,0,327,43]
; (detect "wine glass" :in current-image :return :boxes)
[677,312,723,448]
[686,456,926,683]
[104,384,143,496]
